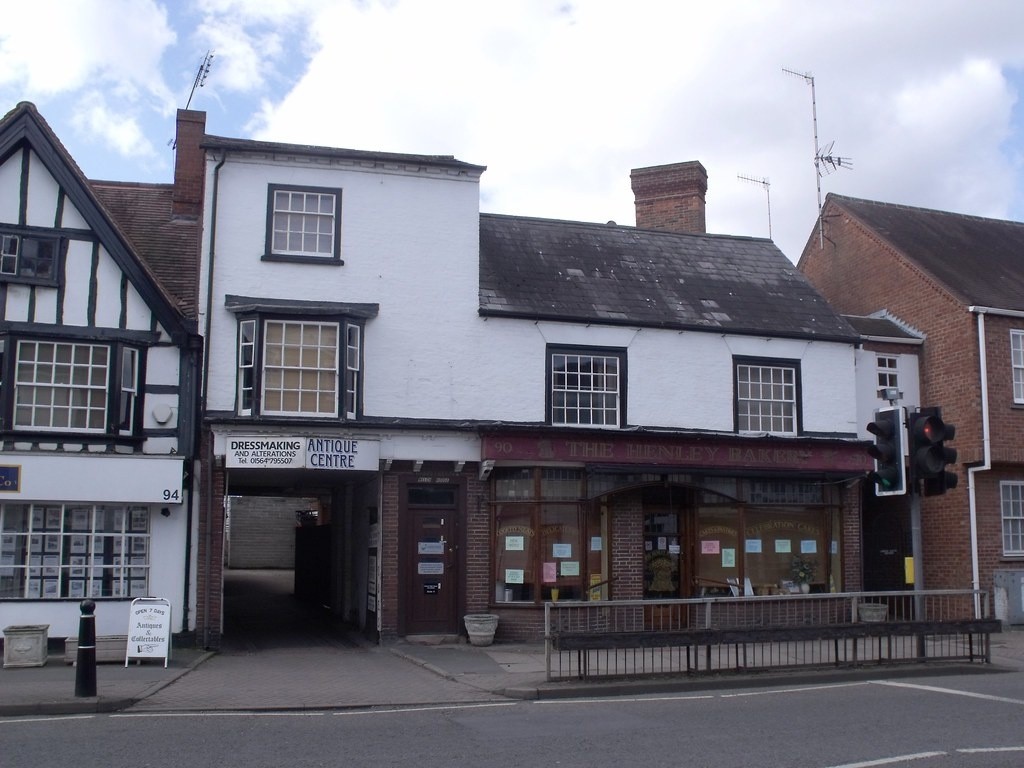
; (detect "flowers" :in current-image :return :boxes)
[791,555,817,585]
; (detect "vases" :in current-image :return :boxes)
[65,636,128,666]
[800,583,809,594]
[857,603,888,622]
[2,624,51,668]
[463,614,499,647]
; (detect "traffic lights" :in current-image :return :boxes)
[909,410,947,496]
[866,404,906,497]
[922,405,958,494]
